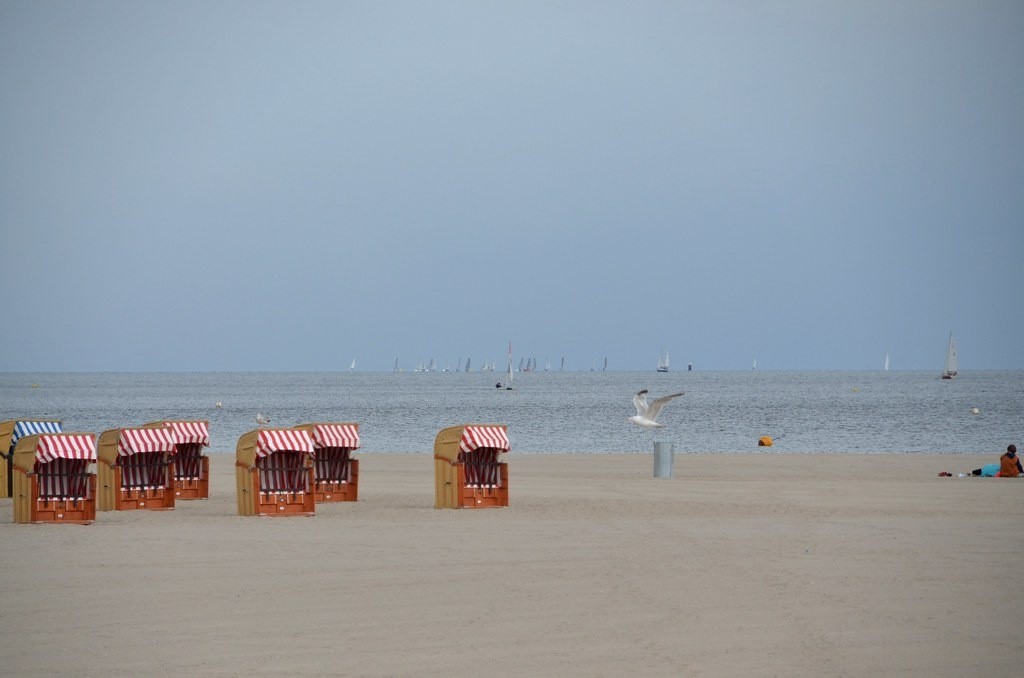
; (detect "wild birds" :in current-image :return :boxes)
[624,389,686,430]
[255,412,270,428]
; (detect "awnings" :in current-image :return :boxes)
[11,421,64,448]
[311,424,361,451]
[35,435,97,464]
[117,429,177,458]
[459,425,510,453]
[256,430,315,459]
[164,422,210,446]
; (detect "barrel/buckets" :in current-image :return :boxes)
[654,440,674,479]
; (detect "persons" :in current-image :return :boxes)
[999,444,1024,477]
